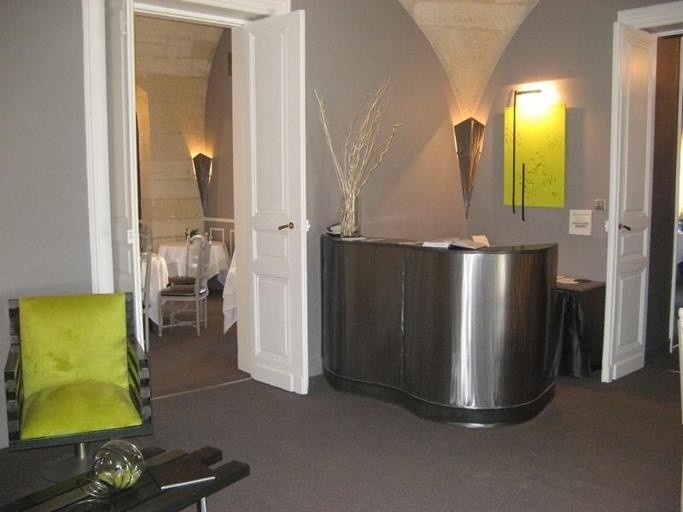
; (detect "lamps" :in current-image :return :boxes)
[193,153,211,216]
[454,118,485,218]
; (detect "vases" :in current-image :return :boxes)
[339,195,362,238]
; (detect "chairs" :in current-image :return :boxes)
[4,292,153,483]
[139,219,211,354]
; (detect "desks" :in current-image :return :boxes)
[159,241,231,296]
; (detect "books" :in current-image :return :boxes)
[422,236,486,249]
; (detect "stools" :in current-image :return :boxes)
[556,279,605,378]
